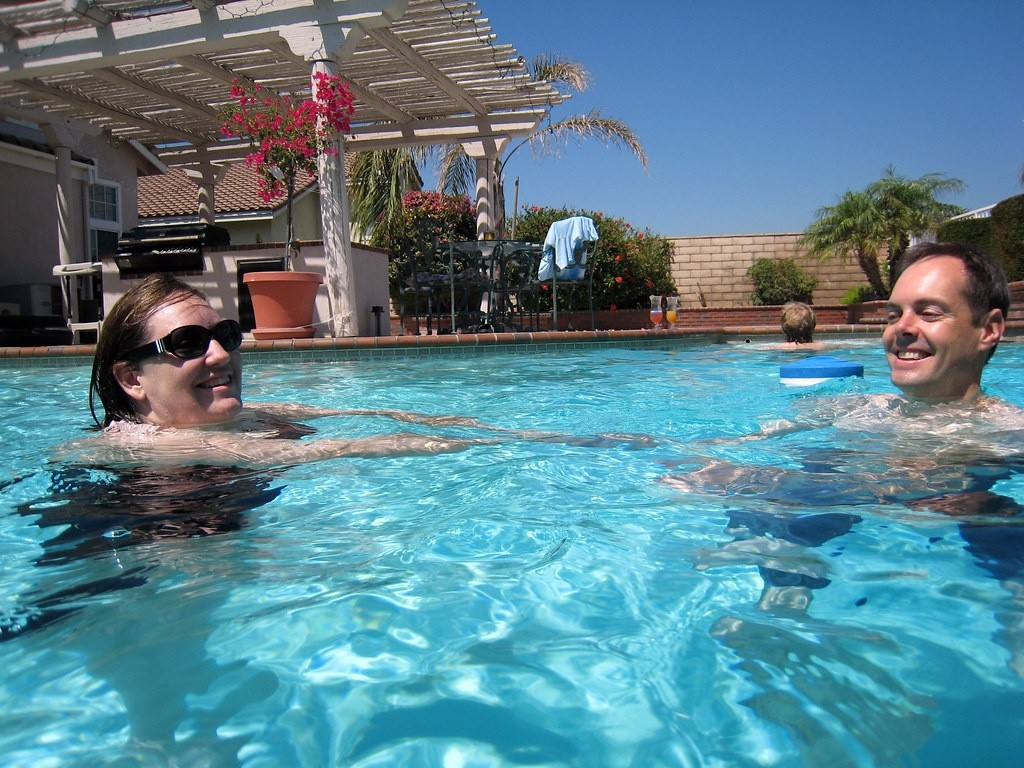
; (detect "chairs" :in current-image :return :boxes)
[393,216,598,335]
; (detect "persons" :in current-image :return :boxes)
[755,301,833,350]
[765,239,1023,470]
[39,271,658,546]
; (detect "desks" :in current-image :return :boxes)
[433,239,546,334]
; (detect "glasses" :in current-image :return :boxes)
[118,319,244,362]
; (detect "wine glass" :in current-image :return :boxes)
[666,297,680,330]
[650,296,663,330]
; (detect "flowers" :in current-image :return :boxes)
[218,70,357,271]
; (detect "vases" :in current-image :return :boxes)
[244,271,324,340]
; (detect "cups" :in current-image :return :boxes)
[484,231,495,240]
